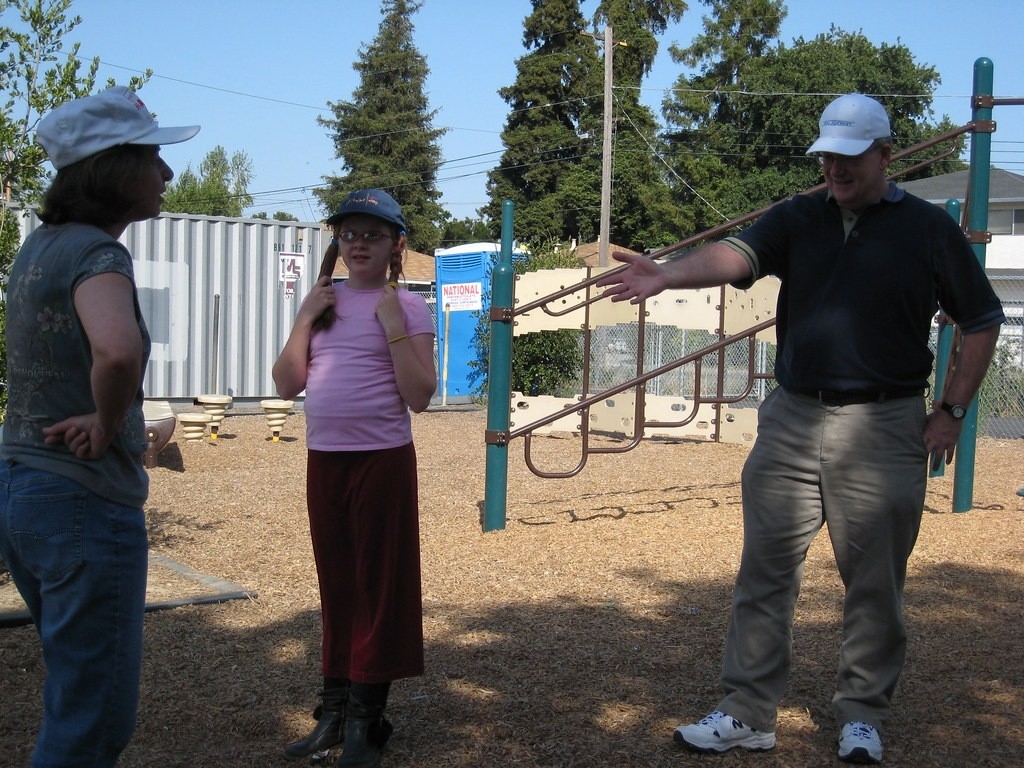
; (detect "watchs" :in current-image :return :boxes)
[938,401,968,418]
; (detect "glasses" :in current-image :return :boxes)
[338,230,392,242]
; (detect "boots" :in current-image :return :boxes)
[283,678,350,756]
[332,683,392,768]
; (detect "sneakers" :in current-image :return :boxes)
[837,721,883,761]
[673,709,777,753]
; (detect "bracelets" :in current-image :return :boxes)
[387,334,408,343]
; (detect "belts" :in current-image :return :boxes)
[801,387,924,405]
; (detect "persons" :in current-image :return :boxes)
[0,85,200,768]
[597,91,1008,764]
[272,191,439,768]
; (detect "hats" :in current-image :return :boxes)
[325,189,408,235]
[36,85,201,170]
[805,93,891,156]
